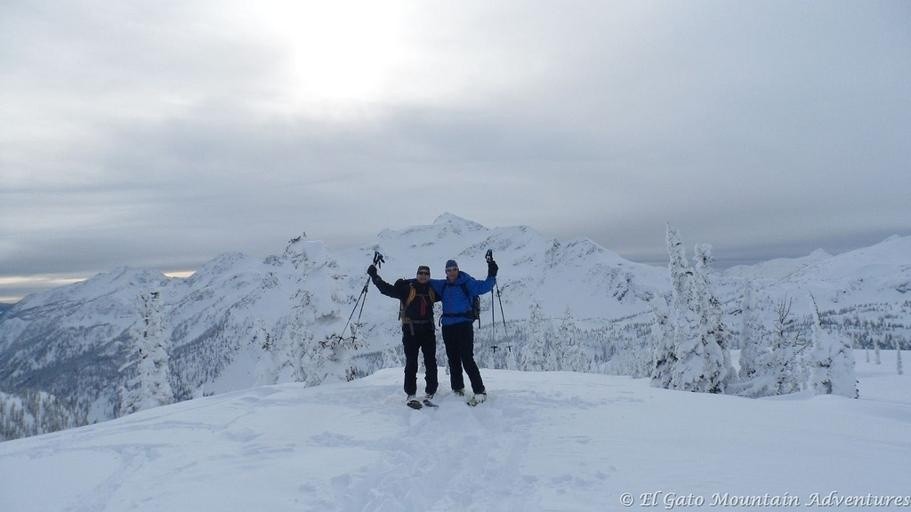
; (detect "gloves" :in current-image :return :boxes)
[367,265,377,277]
[488,261,498,277]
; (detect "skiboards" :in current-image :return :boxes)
[454,390,478,406]
[407,399,438,409]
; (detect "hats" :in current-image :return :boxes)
[417,266,430,271]
[446,260,457,268]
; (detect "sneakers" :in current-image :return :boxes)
[407,394,416,400]
[453,387,465,397]
[425,393,434,401]
[475,390,487,403]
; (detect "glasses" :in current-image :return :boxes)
[447,269,458,271]
[418,272,430,276]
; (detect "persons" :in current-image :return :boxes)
[394,260,498,406]
[367,265,442,409]
